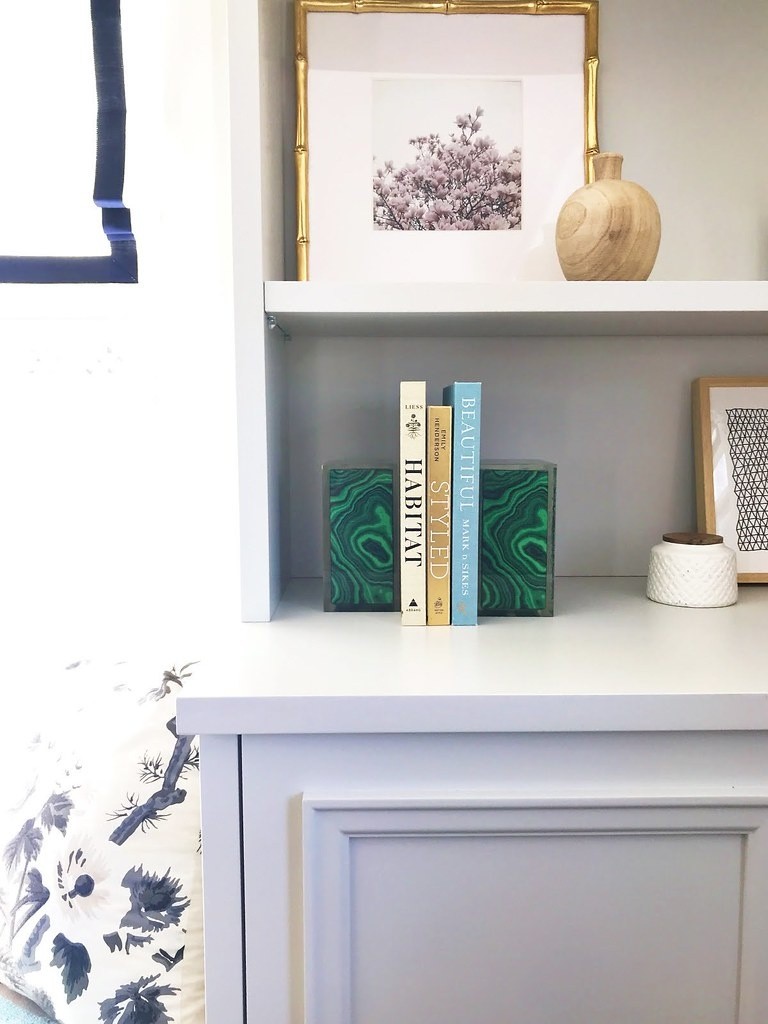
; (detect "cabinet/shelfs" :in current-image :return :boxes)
[176,1,768,1024]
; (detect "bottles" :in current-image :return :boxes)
[556,153,661,282]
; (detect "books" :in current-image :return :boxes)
[398,379,483,629]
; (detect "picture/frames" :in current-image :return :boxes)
[293,0,598,279]
[691,375,768,583]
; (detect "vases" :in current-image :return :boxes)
[557,152,661,281]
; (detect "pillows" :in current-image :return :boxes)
[0,658,205,1024]
